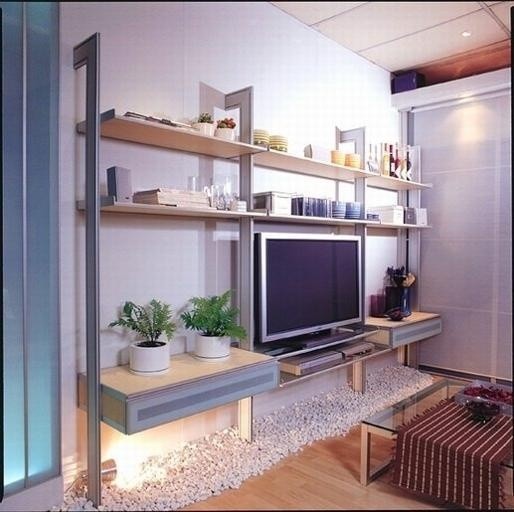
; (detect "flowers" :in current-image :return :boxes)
[218,113,238,129]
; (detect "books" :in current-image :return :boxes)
[130,188,217,210]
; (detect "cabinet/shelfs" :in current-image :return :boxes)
[369,171,435,233]
[101,107,269,228]
[254,145,378,223]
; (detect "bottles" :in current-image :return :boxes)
[366,138,414,183]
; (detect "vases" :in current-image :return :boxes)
[218,130,236,140]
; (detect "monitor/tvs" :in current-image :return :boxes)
[254,232,362,348]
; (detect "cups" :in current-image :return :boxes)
[330,148,361,170]
[290,191,362,219]
[370,293,386,318]
[253,128,288,153]
[187,173,240,212]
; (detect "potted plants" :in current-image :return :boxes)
[180,283,249,360]
[192,109,215,135]
[109,290,179,375]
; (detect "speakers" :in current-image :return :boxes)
[391,71,425,94]
[107,166,133,204]
